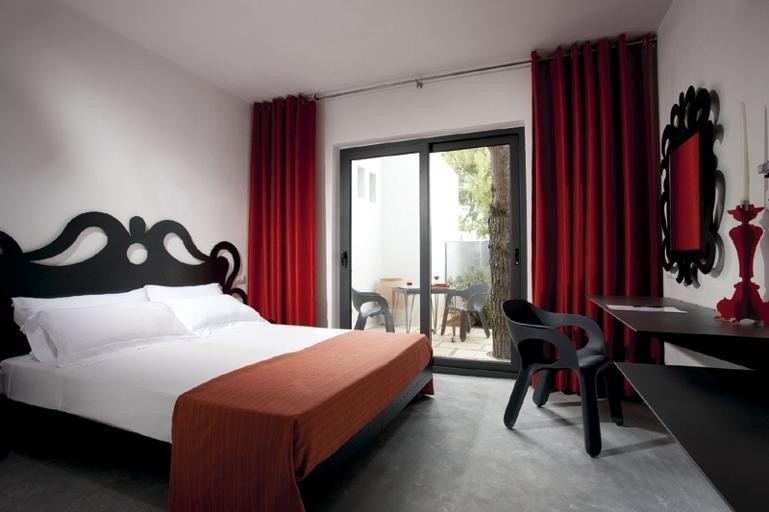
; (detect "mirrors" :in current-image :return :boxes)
[656,84,718,285]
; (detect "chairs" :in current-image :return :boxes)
[441,284,490,342]
[351,287,395,333]
[501,298,623,458]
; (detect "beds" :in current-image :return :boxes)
[0,212,433,511]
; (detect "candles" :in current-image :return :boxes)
[739,100,749,207]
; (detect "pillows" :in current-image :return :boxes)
[12,283,272,369]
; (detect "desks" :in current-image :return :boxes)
[392,284,457,337]
[587,295,769,511]
